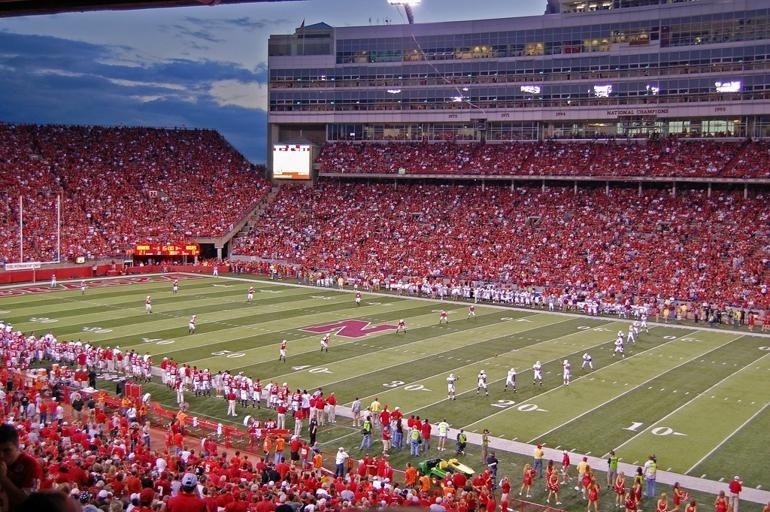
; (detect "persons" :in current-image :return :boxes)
[2,123,769,511]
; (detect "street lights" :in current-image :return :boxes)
[387,0,422,25]
